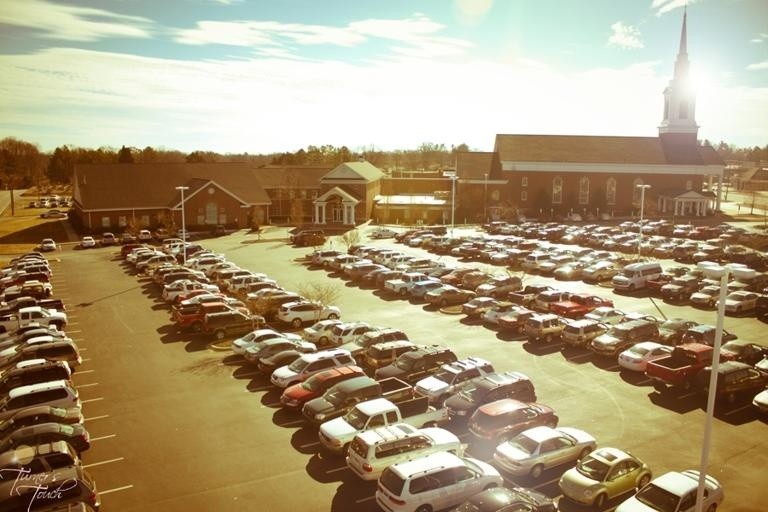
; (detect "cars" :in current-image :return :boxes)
[82,236,95,247]
[41,238,56,251]
[29,194,69,207]
[40,210,66,218]
[103,232,115,244]
[0,252,101,512]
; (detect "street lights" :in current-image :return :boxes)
[175,186,190,265]
[636,184,651,262]
[450,176,459,228]
